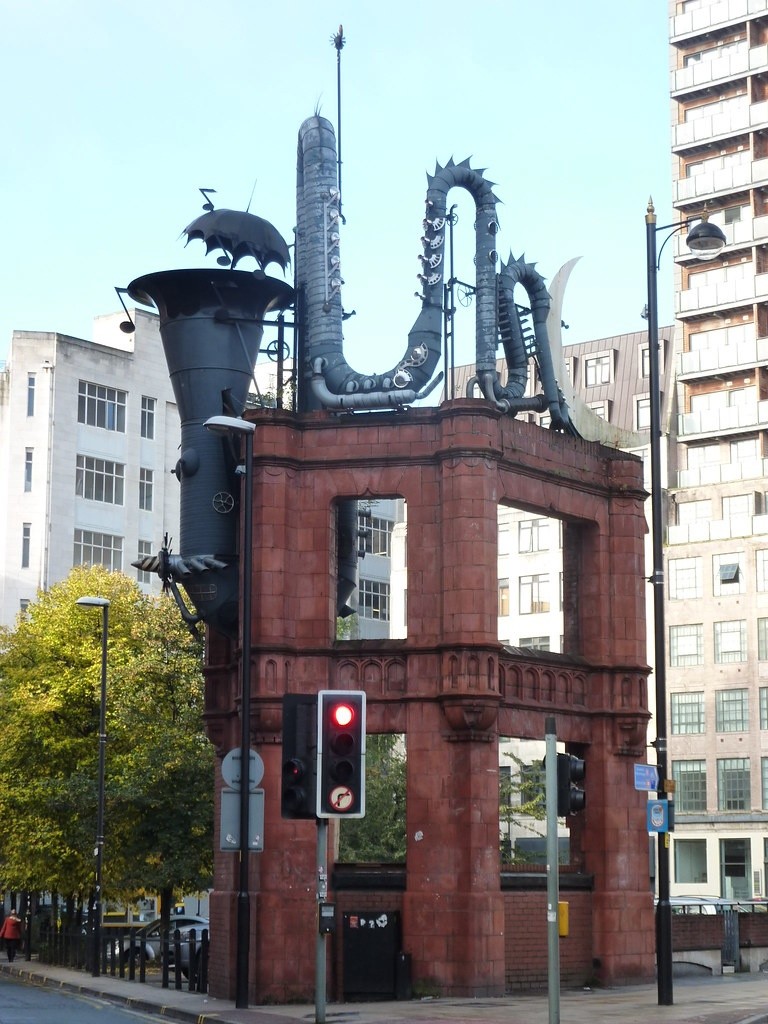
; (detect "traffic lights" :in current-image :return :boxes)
[557,753,587,816]
[278,746,320,822]
[318,692,369,818]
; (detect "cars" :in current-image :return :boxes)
[98,913,210,963]
[653,894,768,918]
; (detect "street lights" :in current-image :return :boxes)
[76,595,111,974]
[204,415,257,1009]
[641,192,729,1004]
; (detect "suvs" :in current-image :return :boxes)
[161,924,211,984]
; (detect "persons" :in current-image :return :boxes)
[0,910,23,963]
